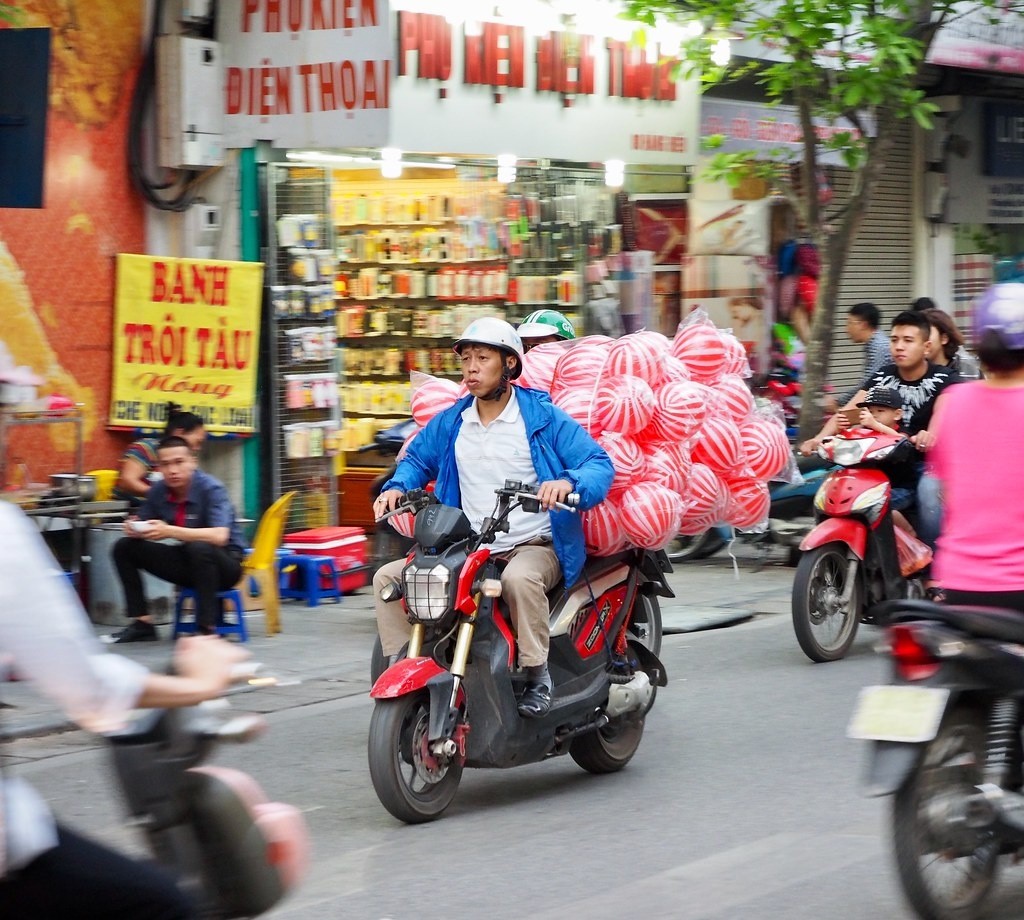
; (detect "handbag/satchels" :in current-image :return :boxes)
[892,524,932,578]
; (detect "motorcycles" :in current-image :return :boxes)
[849,599,1024,920]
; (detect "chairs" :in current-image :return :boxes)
[228,490,296,631]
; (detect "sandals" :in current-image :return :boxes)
[924,586,949,603]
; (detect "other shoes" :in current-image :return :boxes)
[111,619,158,643]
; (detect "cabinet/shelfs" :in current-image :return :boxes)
[0,401,83,588]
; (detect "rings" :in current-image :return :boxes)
[920,444,925,447]
[379,498,387,503]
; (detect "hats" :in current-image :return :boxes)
[856,386,902,410]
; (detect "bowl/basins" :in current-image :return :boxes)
[47,474,78,497]
[74,478,96,501]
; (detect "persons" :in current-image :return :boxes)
[517,309,576,355]
[0,501,254,920]
[111,436,247,644]
[827,302,895,410]
[836,386,921,539]
[800,311,966,603]
[102,402,207,523]
[729,296,764,341]
[374,317,615,719]
[923,282,1024,612]
[923,307,976,380]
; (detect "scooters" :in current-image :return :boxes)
[105,671,308,920]
[369,482,674,825]
[791,425,935,661]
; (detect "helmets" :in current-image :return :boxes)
[970,281,1024,350]
[451,317,524,381]
[517,309,576,341]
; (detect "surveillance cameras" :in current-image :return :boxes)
[953,137,973,159]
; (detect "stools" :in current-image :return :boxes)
[174,589,246,642]
[276,555,342,605]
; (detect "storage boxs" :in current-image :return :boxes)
[245,547,295,593]
[285,528,367,589]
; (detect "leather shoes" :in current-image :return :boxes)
[516,676,556,719]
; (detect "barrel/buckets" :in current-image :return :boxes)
[84,525,179,626]
